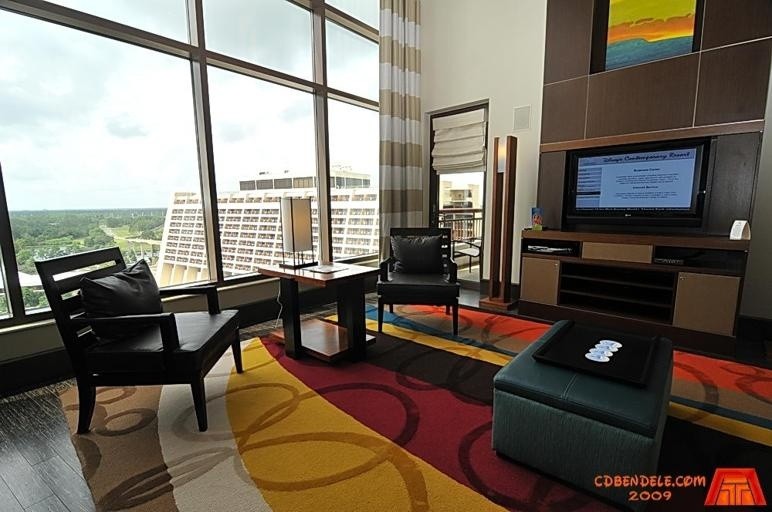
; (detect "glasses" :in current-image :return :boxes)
[279,195,318,270]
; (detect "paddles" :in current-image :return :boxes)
[56,303,772,512]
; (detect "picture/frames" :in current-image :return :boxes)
[258,258,381,364]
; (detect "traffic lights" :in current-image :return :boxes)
[79,258,164,345]
[390,235,444,275]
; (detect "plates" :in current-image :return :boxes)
[517,227,751,359]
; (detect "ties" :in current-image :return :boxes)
[561,136,717,234]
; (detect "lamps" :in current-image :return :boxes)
[33,245,244,434]
[376,227,461,337]
